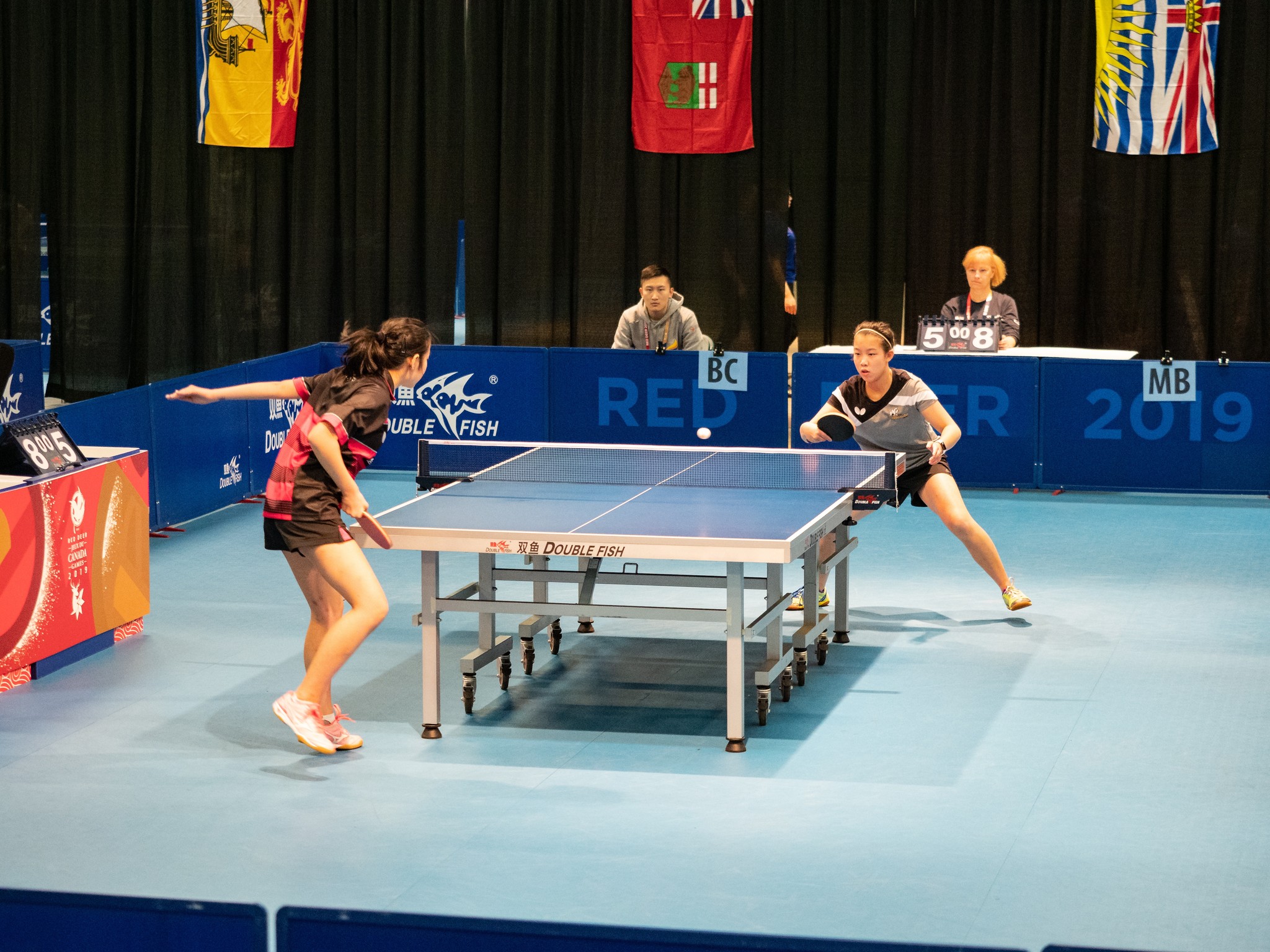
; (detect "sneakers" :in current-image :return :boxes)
[272,690,337,755]
[323,703,364,750]
[785,585,830,610]
[1001,577,1033,611]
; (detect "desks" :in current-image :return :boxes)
[805,344,1141,360]
[346,431,908,761]
[0,444,152,693]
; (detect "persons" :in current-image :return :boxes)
[940,245,1020,351]
[768,188,799,398]
[632,0,755,154]
[785,320,1033,610]
[165,317,436,755]
[611,265,704,351]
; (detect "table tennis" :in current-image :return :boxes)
[697,427,712,440]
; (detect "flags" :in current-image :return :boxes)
[1093,0,1221,155]
[195,0,308,148]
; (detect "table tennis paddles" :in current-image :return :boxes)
[335,487,394,550]
[807,412,856,444]
